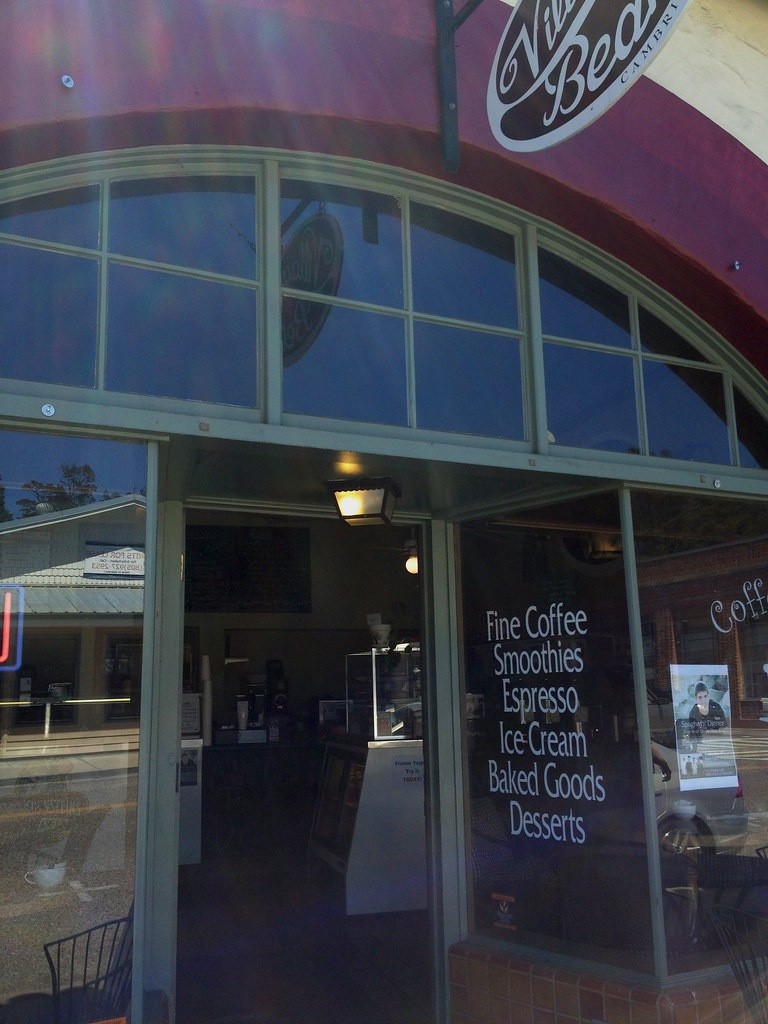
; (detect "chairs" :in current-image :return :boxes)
[705,904,768,1024]
[44,917,135,1024]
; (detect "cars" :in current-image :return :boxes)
[624,739,750,860]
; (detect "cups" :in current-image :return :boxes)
[671,800,697,822]
[24,865,67,888]
[237,708,248,729]
[200,654,214,747]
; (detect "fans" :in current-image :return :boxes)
[361,527,417,561]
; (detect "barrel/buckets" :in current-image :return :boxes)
[370,624,390,648]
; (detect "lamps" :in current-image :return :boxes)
[581,532,624,563]
[405,548,418,575]
[322,476,402,528]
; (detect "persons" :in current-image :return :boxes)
[180,753,197,786]
[603,664,671,845]
[688,682,728,750]
[681,757,687,775]
[685,755,693,776]
[691,757,698,775]
[696,759,705,775]
